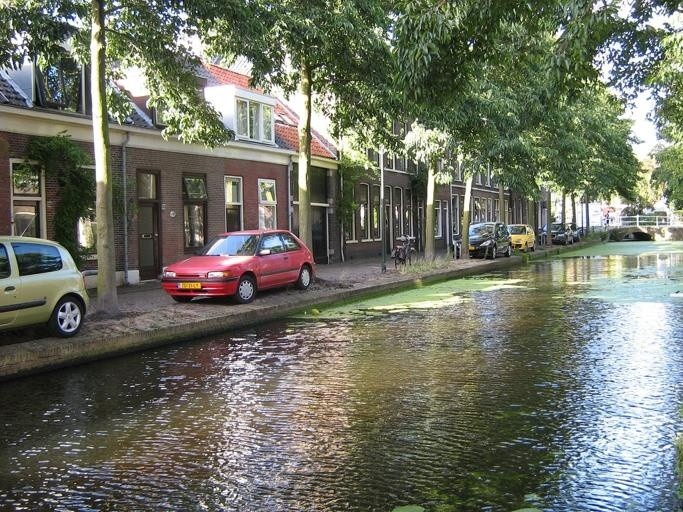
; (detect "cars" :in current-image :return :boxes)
[539,223,591,245]
[0,235,91,338]
[161,229,317,303]
[507,223,536,252]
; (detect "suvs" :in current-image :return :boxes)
[453,222,512,259]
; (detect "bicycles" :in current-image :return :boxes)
[394,235,417,268]
